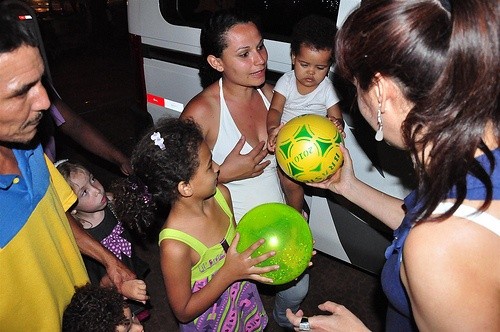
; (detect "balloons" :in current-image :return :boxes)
[234,204,314,286]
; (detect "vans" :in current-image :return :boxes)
[125,0,417,276]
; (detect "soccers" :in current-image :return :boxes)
[276,115,346,182]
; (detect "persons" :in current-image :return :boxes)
[177,8,392,329]
[286,0,500,332]
[0,27,139,332]
[0,1,134,177]
[61,273,150,332]
[111,112,317,332]
[55,162,152,312]
[266,17,345,215]
